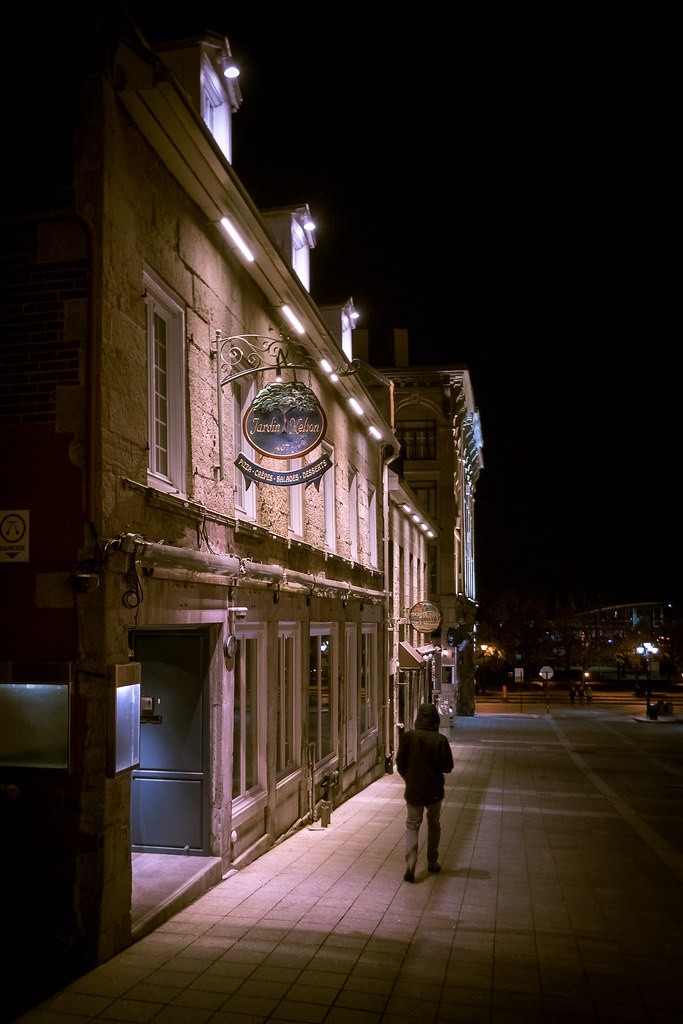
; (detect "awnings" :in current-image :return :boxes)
[399,641,426,671]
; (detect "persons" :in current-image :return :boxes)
[569,683,592,705]
[396,703,455,880]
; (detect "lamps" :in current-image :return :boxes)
[217,55,240,79]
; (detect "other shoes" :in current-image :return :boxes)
[404,866,416,883]
[428,863,441,871]
